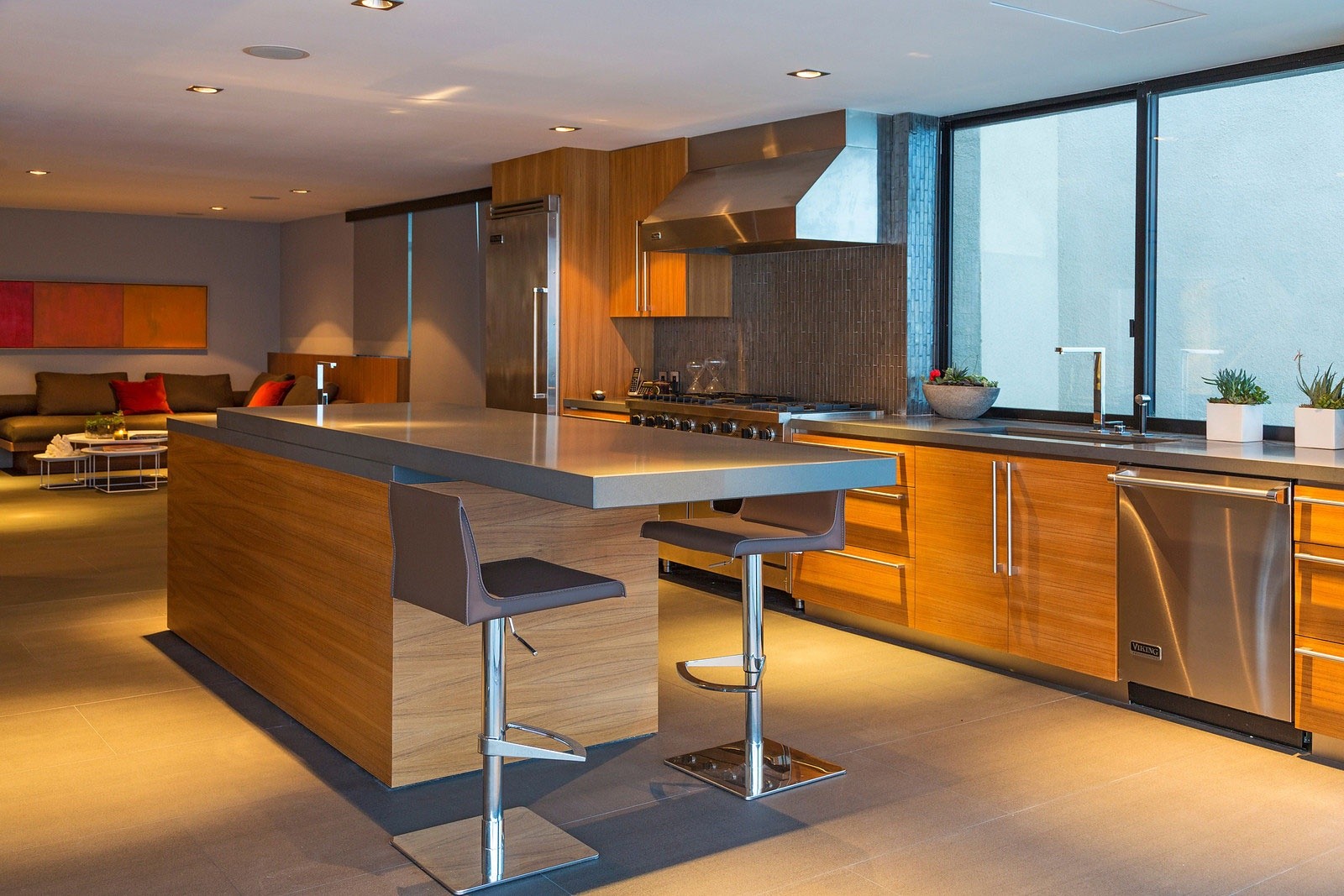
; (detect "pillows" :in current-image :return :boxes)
[34,372,128,415]
[243,371,339,407]
[109,374,174,416]
[145,372,237,414]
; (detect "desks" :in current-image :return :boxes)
[34,430,168,493]
[167,401,896,786]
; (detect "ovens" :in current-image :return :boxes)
[686,498,794,595]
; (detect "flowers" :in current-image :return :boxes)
[920,362,999,387]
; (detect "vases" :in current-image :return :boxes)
[922,384,1000,420]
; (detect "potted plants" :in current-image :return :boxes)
[1295,348,1344,450]
[1201,368,1272,442]
[83,410,126,439]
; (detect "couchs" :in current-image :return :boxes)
[0,391,347,475]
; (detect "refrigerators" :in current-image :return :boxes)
[485,193,560,418]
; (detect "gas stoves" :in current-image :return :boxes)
[625,392,884,442]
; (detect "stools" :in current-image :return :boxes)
[387,480,627,895]
[640,489,846,800]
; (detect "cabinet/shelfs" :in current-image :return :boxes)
[792,430,1344,760]
[610,138,732,318]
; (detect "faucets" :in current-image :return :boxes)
[314,359,337,406]
[1053,345,1110,433]
[1132,393,1154,437]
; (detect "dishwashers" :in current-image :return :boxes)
[1106,464,1295,723]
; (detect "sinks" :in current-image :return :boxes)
[946,425,1183,446]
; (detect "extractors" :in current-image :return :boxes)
[641,108,895,255]
